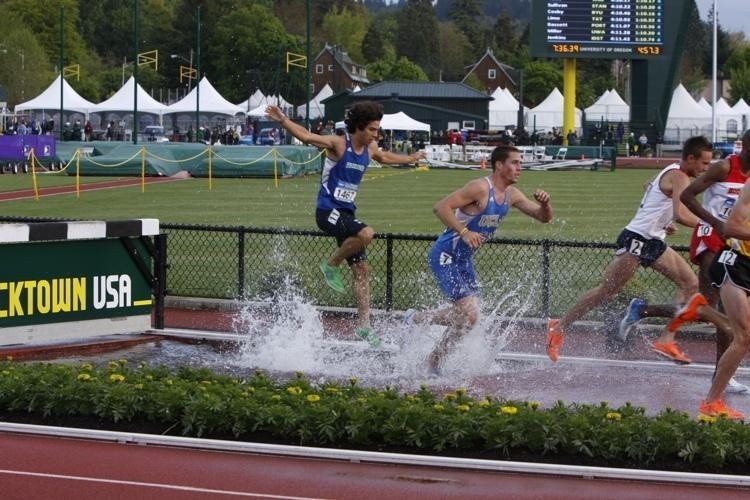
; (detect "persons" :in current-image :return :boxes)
[618,129,750,394]
[264,102,427,348]
[188,125,241,145]
[547,136,714,365]
[399,146,553,375]
[450,121,648,171]
[674,178,750,422]
[5,117,82,141]
[267,120,446,172]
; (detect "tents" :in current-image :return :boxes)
[334,85,431,149]
[15,74,93,120]
[447,86,529,129]
[160,76,244,129]
[297,83,336,120]
[664,82,750,140]
[89,76,167,145]
[529,86,582,132]
[237,88,294,123]
[584,88,630,122]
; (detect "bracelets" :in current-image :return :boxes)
[460,229,467,236]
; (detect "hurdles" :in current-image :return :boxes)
[0,218,169,349]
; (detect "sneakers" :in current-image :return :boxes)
[353,325,381,348]
[723,377,749,394]
[666,292,709,333]
[401,308,416,330]
[545,318,566,363]
[696,399,745,424]
[318,256,348,296]
[618,296,648,343]
[650,337,693,365]
